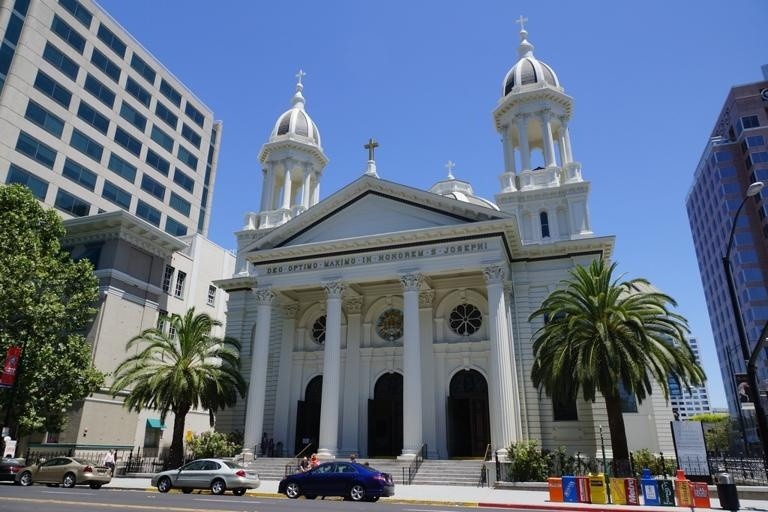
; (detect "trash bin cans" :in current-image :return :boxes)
[715,472,741,510]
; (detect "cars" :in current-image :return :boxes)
[151,458,260,496]
[0,456,112,488]
[278,461,395,502]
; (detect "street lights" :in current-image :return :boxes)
[722,181,765,455]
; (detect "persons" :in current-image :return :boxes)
[313,457,321,467]
[365,461,371,466]
[350,454,358,463]
[310,453,317,468]
[36,454,46,463]
[103,448,115,472]
[296,456,311,474]
[260,432,269,456]
[268,438,275,457]
